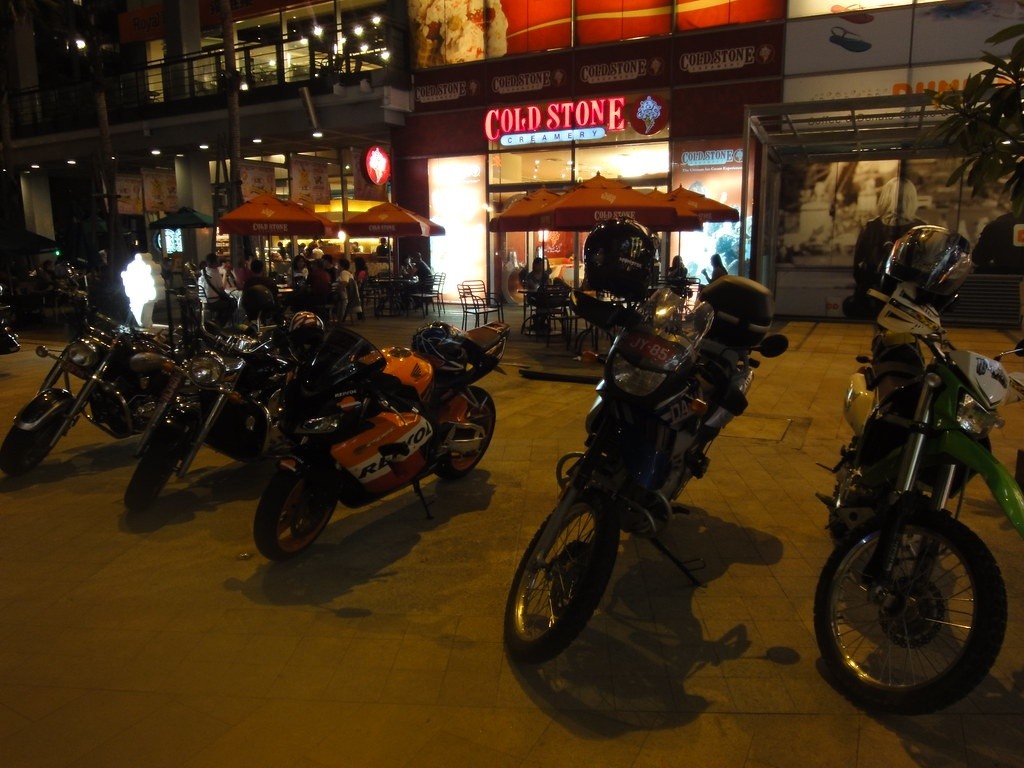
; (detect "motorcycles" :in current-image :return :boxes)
[0,247,294,521]
[252,313,511,559]
[497,275,790,665]
[811,287,1023,715]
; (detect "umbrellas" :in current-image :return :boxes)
[342,202,446,277]
[218,194,324,272]
[490,176,736,285]
[285,199,338,256]
[148,207,213,248]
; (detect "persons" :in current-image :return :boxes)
[524,258,552,323]
[198,252,277,332]
[841,176,928,318]
[376,238,392,256]
[293,255,369,324]
[402,252,433,312]
[667,256,688,286]
[702,254,728,283]
[277,237,325,261]
[352,242,360,252]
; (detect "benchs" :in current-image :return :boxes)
[941,274,1024,328]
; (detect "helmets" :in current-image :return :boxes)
[289,311,323,362]
[583,215,653,299]
[885,224,973,299]
[412,320,468,370]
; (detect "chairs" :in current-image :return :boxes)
[186,271,572,349]
